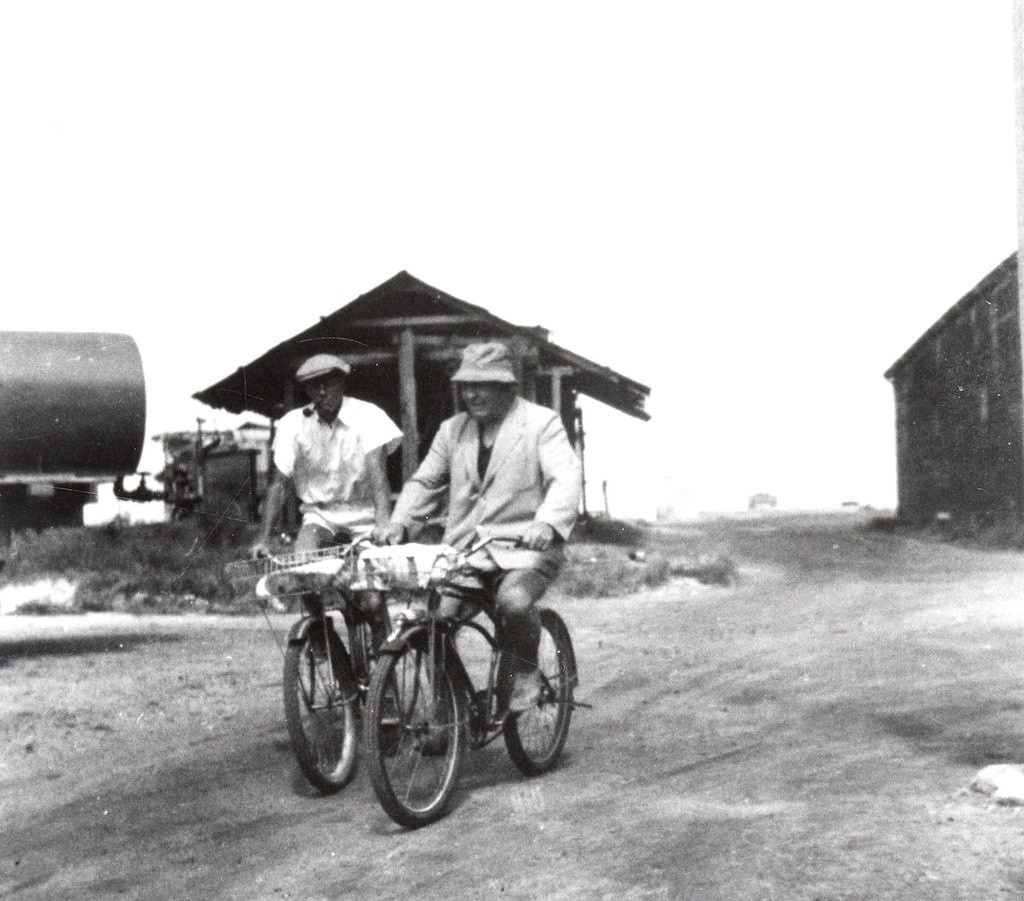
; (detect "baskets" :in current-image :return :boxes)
[225,544,353,604]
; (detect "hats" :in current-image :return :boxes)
[295,353,350,382]
[450,342,518,382]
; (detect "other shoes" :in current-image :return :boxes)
[378,694,399,725]
[510,668,540,712]
[305,617,333,665]
[415,725,450,756]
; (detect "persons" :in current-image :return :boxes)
[247,353,405,695]
[370,342,581,753]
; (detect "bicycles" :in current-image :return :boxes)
[228,533,594,830]
